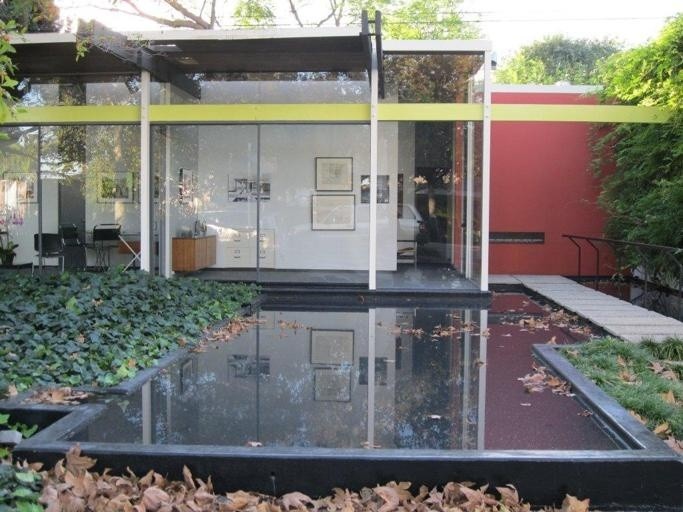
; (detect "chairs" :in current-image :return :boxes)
[31,223,121,273]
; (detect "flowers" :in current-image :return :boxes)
[1,211,23,263]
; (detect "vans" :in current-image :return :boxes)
[313,202,424,257]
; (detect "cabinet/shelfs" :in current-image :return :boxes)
[172,235,217,276]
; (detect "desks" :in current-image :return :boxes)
[119,233,139,272]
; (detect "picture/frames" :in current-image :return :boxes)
[179,168,193,204]
[96,171,134,204]
[312,195,355,231]
[226,228,275,270]
[3,172,38,203]
[315,157,353,191]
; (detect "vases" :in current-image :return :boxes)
[2,253,13,265]
